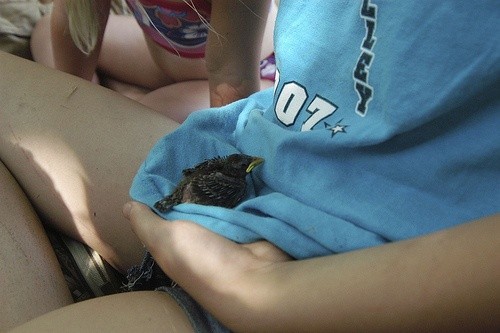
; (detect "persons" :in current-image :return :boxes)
[30,0,278,124]
[0,0,500,333]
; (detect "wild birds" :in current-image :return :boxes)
[154,153,265,215]
